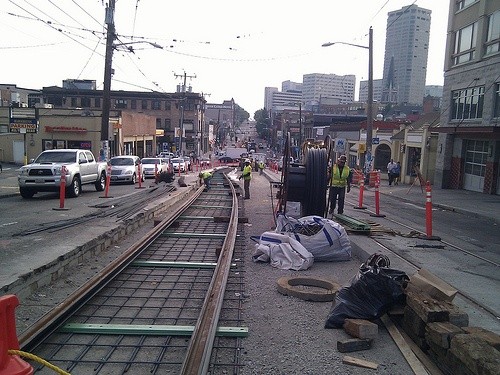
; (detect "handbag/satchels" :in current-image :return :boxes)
[249,233,314,270]
[275,215,352,262]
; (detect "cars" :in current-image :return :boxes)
[140,157,169,179]
[200,158,210,166]
[170,157,187,173]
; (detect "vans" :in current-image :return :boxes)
[159,152,174,159]
[107,155,146,185]
[279,156,294,171]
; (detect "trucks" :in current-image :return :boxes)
[218,147,248,163]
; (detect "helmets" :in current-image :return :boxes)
[244,159,251,164]
[337,155,347,162]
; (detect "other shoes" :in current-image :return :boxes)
[329,209,334,214]
[242,196,250,199]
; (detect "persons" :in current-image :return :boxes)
[327,154,351,215]
[197,156,401,200]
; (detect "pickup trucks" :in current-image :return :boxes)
[18,149,107,199]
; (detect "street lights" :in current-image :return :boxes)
[98,40,163,160]
[283,102,302,145]
[322,28,373,186]
